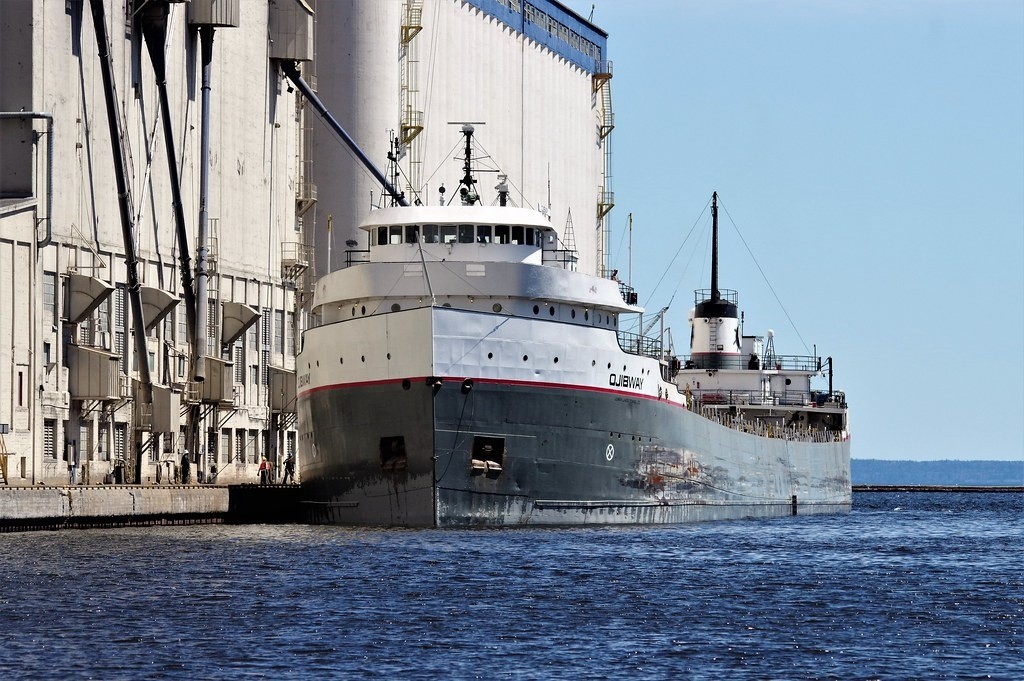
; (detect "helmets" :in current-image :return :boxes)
[262,457,266,461]
[183,450,189,455]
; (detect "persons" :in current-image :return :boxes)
[181,449,190,484]
[257,458,268,485]
[282,452,296,485]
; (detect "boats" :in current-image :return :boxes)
[295,119,853,530]
